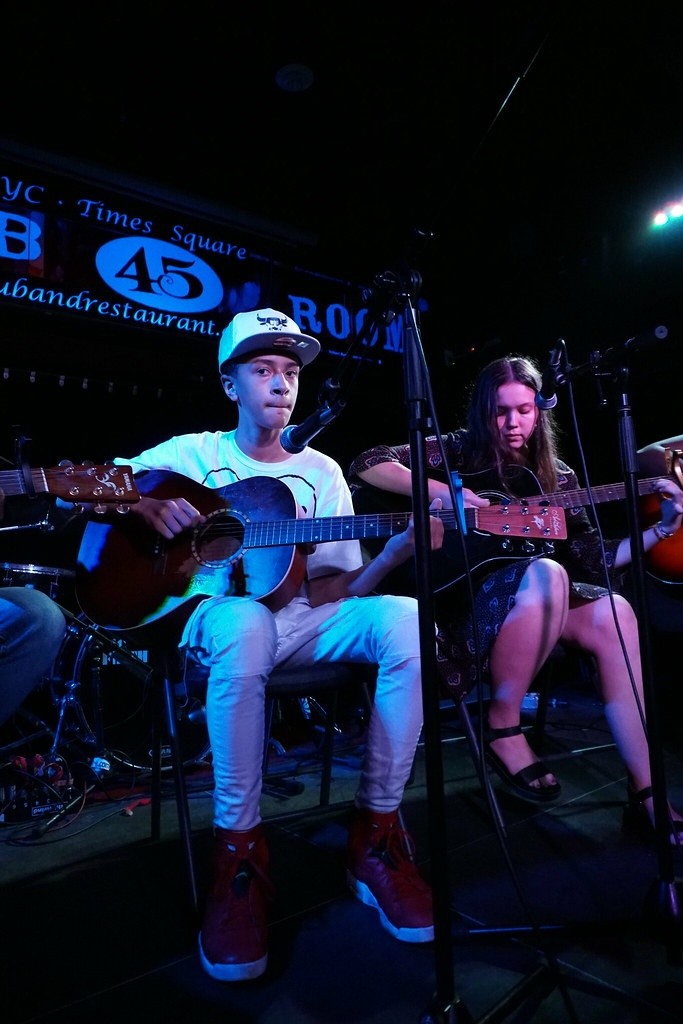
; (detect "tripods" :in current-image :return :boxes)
[30,600,182,838]
[477,327,683,1024]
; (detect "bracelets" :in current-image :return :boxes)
[652,521,675,541]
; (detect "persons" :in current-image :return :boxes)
[56,308,440,983]
[347,356,683,846]
[635,432,683,474]
[0,457,64,737]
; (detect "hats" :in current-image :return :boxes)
[217,308,321,375]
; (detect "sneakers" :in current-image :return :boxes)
[347,816,435,944]
[199,835,271,982]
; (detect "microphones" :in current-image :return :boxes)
[534,338,565,411]
[282,400,347,454]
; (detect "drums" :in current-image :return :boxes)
[2,560,80,615]
[47,618,213,774]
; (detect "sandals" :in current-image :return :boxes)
[623,782,683,852]
[475,717,561,801]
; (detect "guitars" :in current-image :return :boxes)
[350,446,683,609]
[71,464,571,634]
[603,454,683,587]
[1,458,144,518]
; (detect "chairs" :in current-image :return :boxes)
[433,642,592,801]
[145,621,375,957]
